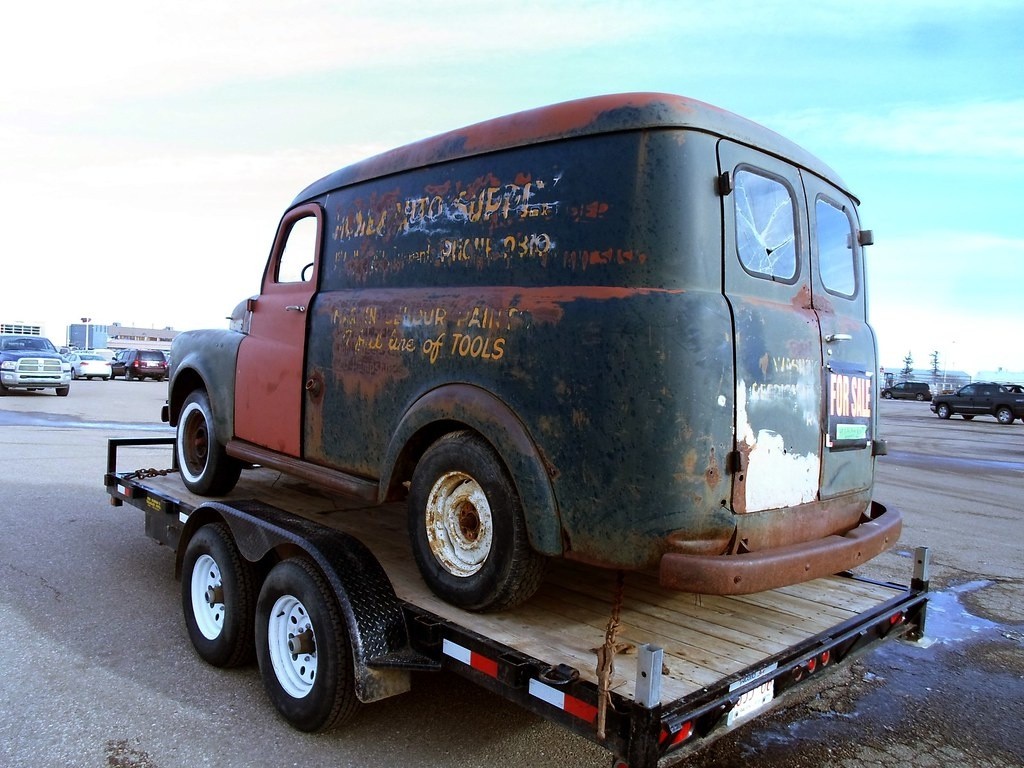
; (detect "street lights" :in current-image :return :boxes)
[80,317,92,350]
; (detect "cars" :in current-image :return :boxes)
[159,93,902,615]
[58,347,79,360]
[93,349,121,359]
[881,382,932,402]
[66,352,112,381]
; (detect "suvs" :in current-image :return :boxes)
[0,333,71,396]
[111,349,168,382]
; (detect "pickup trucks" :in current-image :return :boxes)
[930,382,1024,425]
